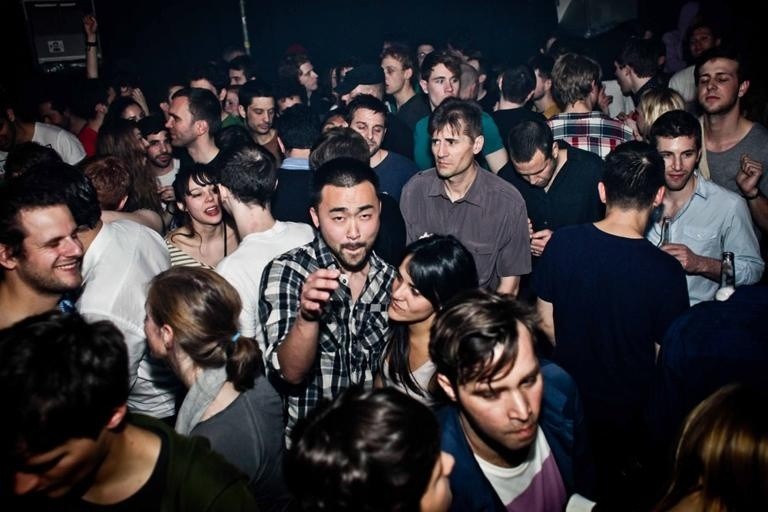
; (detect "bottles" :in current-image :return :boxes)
[713,251,737,304]
[657,215,675,251]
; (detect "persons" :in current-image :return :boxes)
[1,15,767,511]
